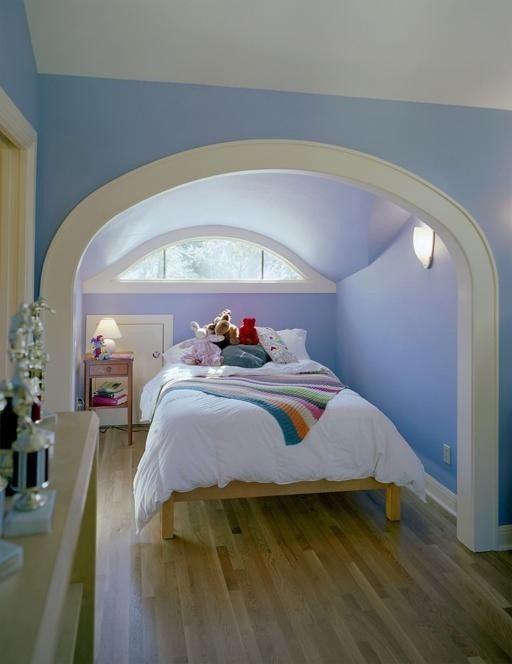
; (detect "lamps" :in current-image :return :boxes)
[412,225,437,270]
[93,317,122,356]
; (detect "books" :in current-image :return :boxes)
[110,352,135,359]
[90,380,128,406]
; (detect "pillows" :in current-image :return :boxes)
[254,326,300,364]
[160,337,198,365]
[276,329,312,362]
[220,344,268,369]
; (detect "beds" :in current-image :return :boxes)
[132,326,431,541]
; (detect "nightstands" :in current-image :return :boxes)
[83,352,134,448]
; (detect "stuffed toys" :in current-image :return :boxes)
[90,334,110,359]
[181,308,260,365]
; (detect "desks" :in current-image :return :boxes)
[1,410,102,663]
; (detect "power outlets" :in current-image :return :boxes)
[441,443,451,467]
